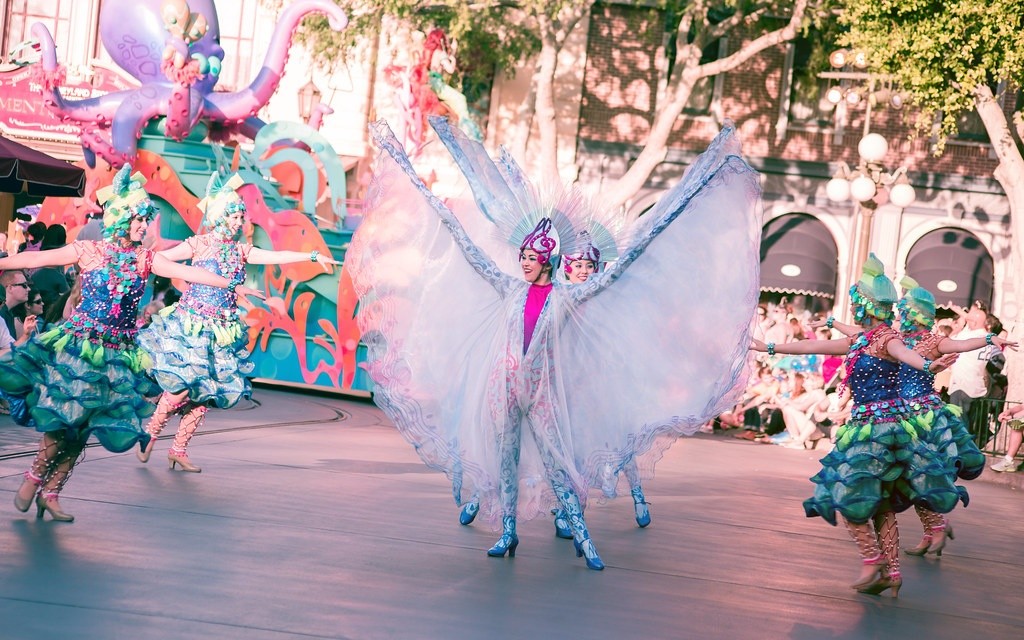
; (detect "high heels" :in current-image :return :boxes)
[486,534,520,557]
[167,453,202,472]
[850,557,892,590]
[135,442,150,463]
[573,536,605,570]
[905,541,943,558]
[926,521,956,556]
[633,502,653,528]
[553,516,576,538]
[34,494,75,521]
[855,577,904,598]
[13,481,36,513]
[460,505,477,525]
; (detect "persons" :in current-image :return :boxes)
[0,163,267,522]
[135,171,341,473]
[750,254,960,599]
[692,297,1024,473]
[346,115,766,572]
[808,289,1020,558]
[0,222,183,426]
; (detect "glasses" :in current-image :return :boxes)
[31,298,43,305]
[758,313,766,317]
[12,281,28,289]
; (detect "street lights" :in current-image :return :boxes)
[825,132,916,326]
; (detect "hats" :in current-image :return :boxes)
[95,161,154,232]
[195,171,247,229]
[898,275,938,333]
[847,252,899,327]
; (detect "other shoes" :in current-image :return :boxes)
[989,459,1017,473]
[734,430,756,440]
[699,427,713,434]
[720,421,732,431]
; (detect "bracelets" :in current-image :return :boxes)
[311,250,319,262]
[826,316,835,328]
[228,279,242,293]
[922,360,937,376]
[985,333,997,344]
[767,342,775,356]
